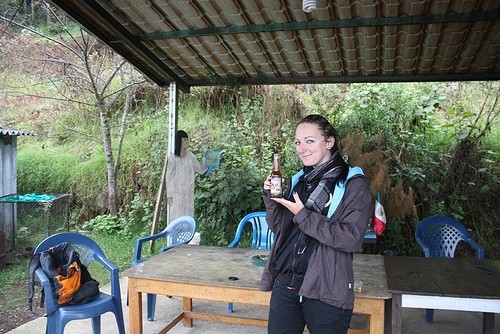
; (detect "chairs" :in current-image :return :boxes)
[227,212,274,313]
[415,216,485,322]
[32,232,125,334]
[126,215,196,321]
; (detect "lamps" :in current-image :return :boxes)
[302,0,317,13]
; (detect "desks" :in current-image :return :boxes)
[383,255,500,334]
[125,243,393,334]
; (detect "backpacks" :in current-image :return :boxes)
[28,242,100,317]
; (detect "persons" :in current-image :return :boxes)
[165,130,207,233]
[260,114,375,334]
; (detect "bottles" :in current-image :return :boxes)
[271,154,283,198]
